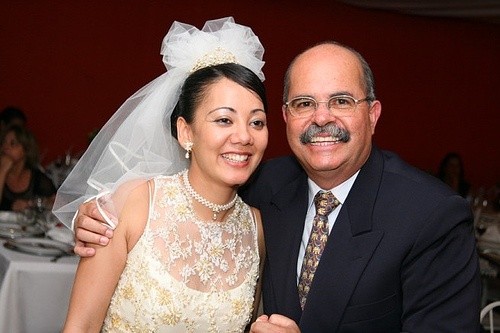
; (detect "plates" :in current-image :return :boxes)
[14,237,75,256]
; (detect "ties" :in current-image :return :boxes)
[296,191,340,309]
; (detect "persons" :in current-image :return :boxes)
[63,18,271,333]
[73,46,482,333]
[0,106,66,212]
[439,152,470,199]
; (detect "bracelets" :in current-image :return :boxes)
[36,197,42,211]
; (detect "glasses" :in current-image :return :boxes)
[285,94,374,118]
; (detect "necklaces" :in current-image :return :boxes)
[183,169,235,219]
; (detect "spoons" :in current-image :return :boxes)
[51,238,75,263]
